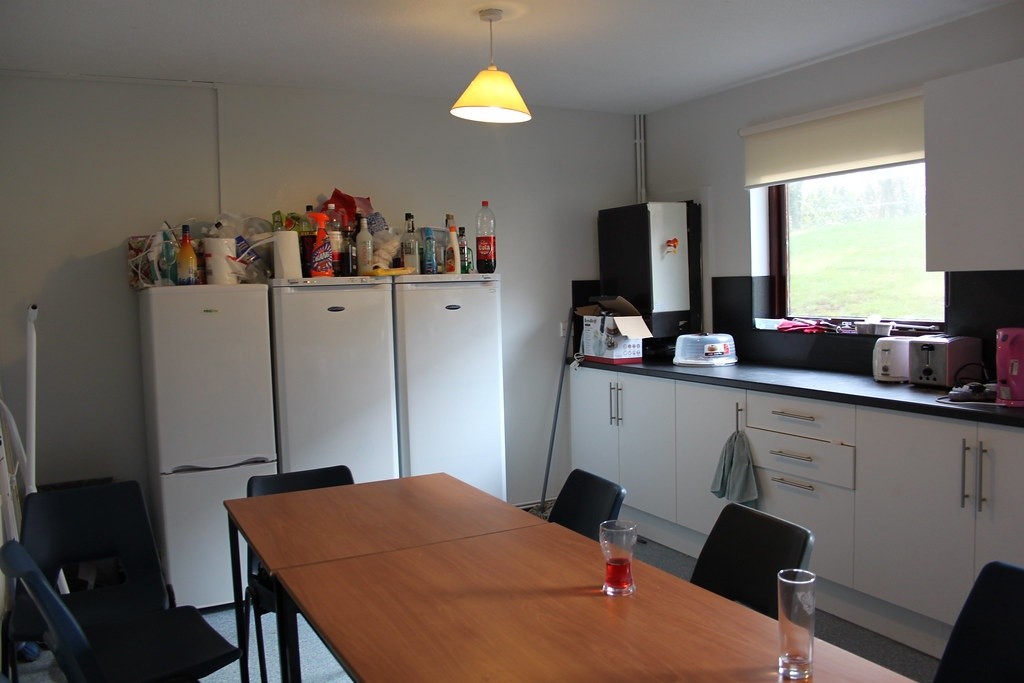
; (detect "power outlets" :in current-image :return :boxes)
[560,321,574,337]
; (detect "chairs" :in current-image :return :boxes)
[690,502,815,621]
[237,463,354,683]
[0,539,241,682]
[2,481,176,682]
[934,561,1024,683]
[547,468,626,543]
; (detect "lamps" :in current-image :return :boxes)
[451,9,534,126]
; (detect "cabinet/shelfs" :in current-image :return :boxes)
[856,395,1023,657]
[678,373,746,559]
[923,58,1024,272]
[566,360,678,551]
[746,380,856,625]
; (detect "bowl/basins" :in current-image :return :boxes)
[855,321,894,335]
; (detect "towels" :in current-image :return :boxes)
[709,430,760,510]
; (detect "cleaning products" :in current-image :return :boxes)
[307,212,334,278]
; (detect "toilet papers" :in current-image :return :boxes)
[272,231,302,278]
[203,237,237,285]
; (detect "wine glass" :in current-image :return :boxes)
[157,240,178,286]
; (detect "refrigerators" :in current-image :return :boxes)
[135,283,279,609]
[394,272,507,502]
[264,276,400,487]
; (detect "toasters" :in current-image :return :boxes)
[872,336,918,382]
[908,333,983,387]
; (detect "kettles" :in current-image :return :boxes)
[996,327,1024,407]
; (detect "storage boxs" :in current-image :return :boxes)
[574,296,653,365]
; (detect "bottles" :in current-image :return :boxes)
[401,212,421,275]
[421,226,445,273]
[176,225,197,286]
[300,203,373,277]
[444,213,474,274]
[475,201,496,273]
[214,221,273,284]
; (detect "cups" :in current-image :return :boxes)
[776,568,817,680]
[598,520,638,596]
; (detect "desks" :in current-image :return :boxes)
[222,471,914,683]
